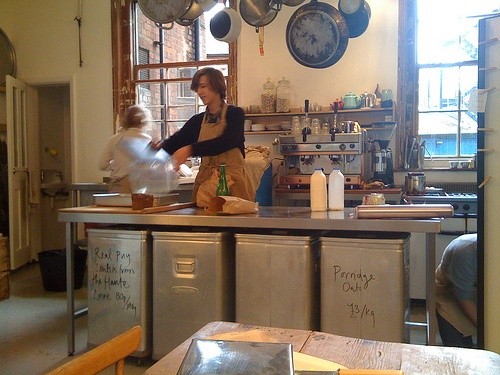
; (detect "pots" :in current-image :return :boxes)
[135,0,371,70]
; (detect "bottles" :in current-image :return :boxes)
[362,193,385,206]
[309,168,328,212]
[374,84,381,98]
[327,167,344,212]
[216,161,228,197]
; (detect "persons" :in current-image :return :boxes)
[151,67,245,206]
[97,105,176,194]
[435,234,477,348]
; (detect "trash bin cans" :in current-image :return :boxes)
[38,248,88,292]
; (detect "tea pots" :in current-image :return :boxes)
[329,98,344,111]
[341,91,361,110]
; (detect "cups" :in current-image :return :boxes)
[293,115,321,135]
[380,89,394,108]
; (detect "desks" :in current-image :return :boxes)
[143,319,500,375]
[57,203,444,355]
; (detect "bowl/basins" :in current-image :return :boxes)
[450,162,471,168]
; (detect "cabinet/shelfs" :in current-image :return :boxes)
[243,102,398,134]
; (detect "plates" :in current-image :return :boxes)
[244,119,281,132]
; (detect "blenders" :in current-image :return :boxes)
[369,122,397,188]
